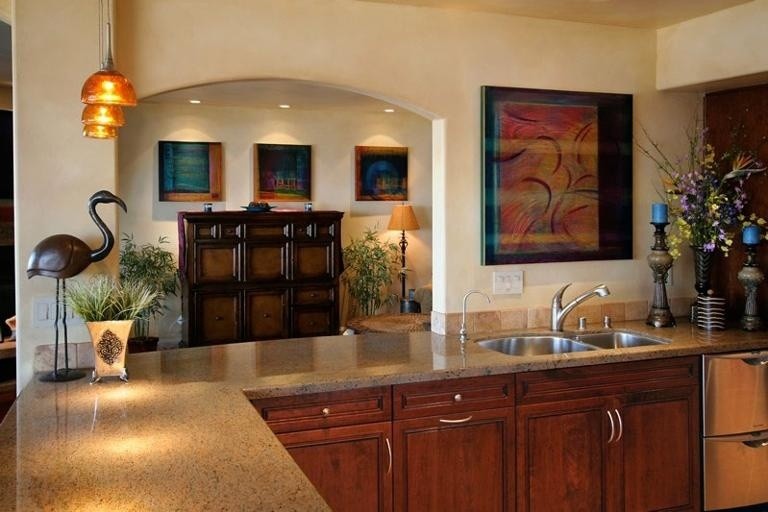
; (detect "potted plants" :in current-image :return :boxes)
[117,231,181,354]
[56,276,168,386]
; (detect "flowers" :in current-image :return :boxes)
[623,81,767,264]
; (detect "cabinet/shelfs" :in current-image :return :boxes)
[390,375,513,512]
[513,354,702,512]
[176,211,345,349]
[243,381,391,512]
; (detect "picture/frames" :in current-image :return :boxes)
[480,84,634,266]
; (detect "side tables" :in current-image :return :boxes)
[346,312,432,333]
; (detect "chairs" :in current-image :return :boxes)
[414,283,432,314]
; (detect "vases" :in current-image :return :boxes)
[690,243,718,293]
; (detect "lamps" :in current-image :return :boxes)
[80,0,137,141]
[386,201,420,315]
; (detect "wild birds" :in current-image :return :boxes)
[26,190,128,376]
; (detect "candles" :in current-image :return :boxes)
[743,225,762,242]
[651,203,668,225]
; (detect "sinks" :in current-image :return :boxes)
[569,325,676,351]
[471,331,602,358]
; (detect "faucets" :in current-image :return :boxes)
[457,288,493,347]
[549,283,612,331]
